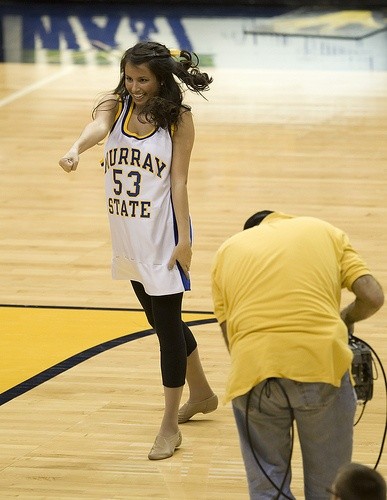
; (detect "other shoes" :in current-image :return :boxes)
[149,428,182,461]
[178,393,218,423]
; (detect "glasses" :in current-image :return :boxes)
[325,487,342,499]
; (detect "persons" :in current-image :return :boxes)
[211,209,385,500]
[58,43,219,461]
[322,461,387,500]
